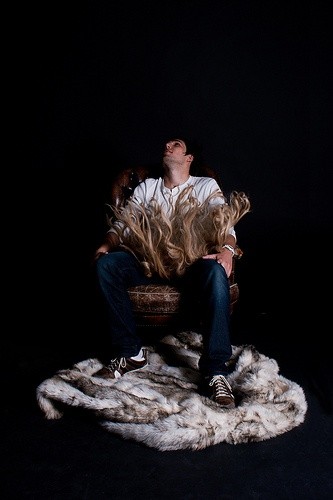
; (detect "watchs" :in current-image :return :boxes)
[222,244,234,253]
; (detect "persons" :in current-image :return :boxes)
[95,137,238,409]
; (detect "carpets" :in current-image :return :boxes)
[37,332,307,452]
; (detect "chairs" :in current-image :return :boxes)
[96,167,243,337]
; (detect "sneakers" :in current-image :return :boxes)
[92,355,148,381]
[208,374,236,409]
